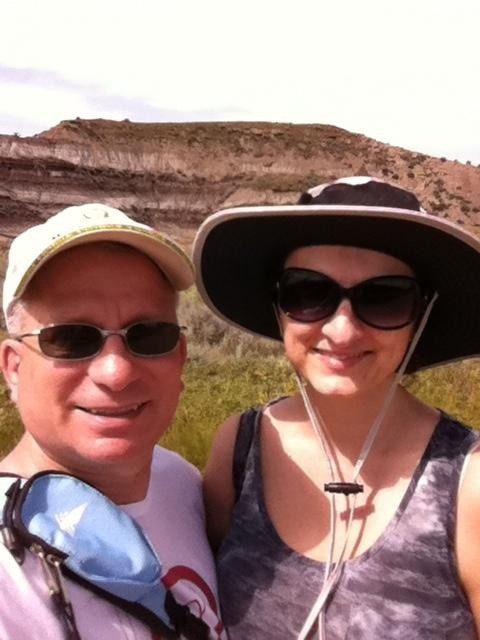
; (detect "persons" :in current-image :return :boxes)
[2,203,229,639]
[192,180,479,638]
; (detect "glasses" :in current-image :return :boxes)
[15,322,188,361]
[274,266,428,330]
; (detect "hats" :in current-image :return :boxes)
[2,203,195,314]
[192,176,480,374]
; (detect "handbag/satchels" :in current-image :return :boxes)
[0,471,162,605]
